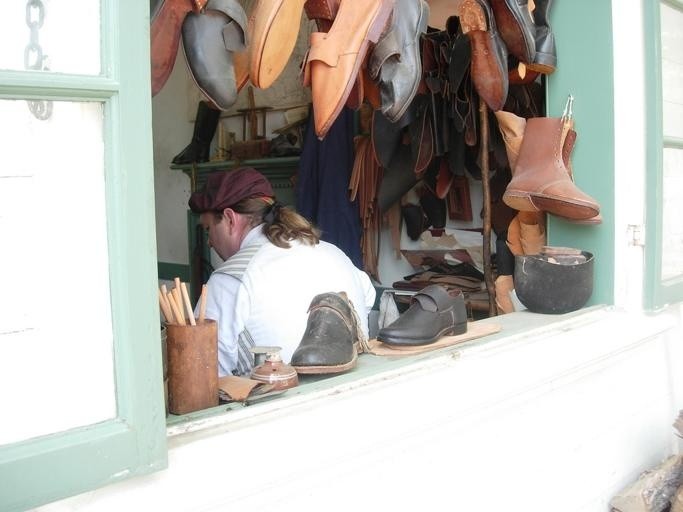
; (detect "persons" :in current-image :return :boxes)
[180,161,376,376]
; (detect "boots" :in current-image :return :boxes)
[172,100,221,164]
[502,116,603,225]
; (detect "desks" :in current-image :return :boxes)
[171,155,302,313]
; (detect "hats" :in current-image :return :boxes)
[189,166,275,213]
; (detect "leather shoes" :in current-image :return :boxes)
[151,0,307,113]
[291,291,366,375]
[377,284,468,346]
[307,0,556,137]
